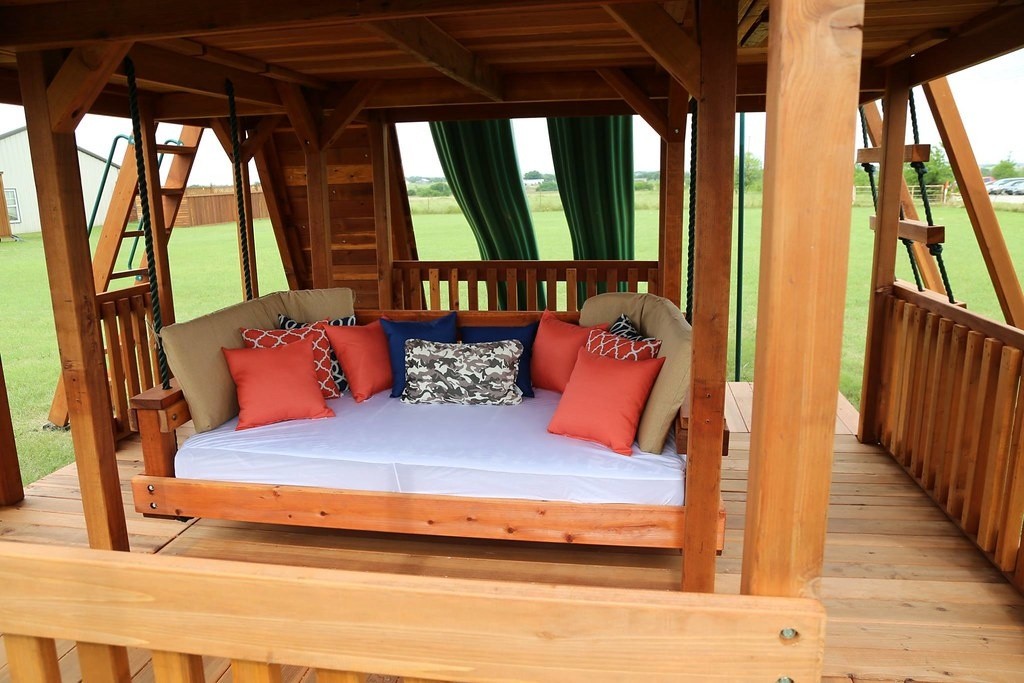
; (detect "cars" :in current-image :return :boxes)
[982,176,1024,195]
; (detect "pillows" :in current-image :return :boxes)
[378,312,457,398]
[610,313,656,341]
[221,335,336,431]
[322,315,393,401]
[238,316,339,401]
[280,313,359,391]
[578,292,691,453]
[584,330,661,360]
[400,339,523,406]
[160,287,353,436]
[530,308,609,394]
[463,322,537,398]
[546,347,667,457]
